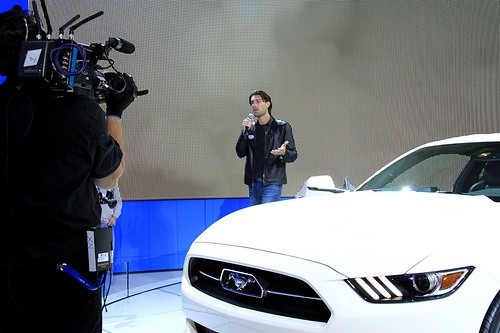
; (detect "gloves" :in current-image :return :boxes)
[103,72,138,121]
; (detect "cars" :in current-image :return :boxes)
[179,134,500,332]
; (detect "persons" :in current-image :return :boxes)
[0,6,139,333]
[235,91,298,205]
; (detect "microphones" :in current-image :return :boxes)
[109,37,135,54]
[244,113,253,134]
